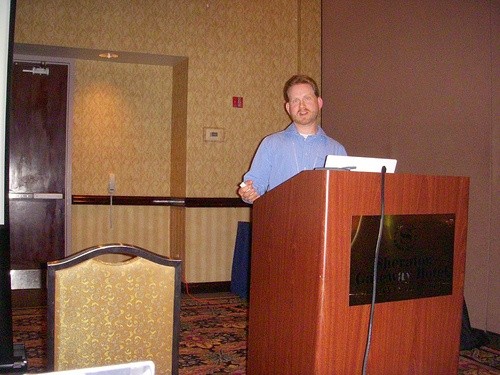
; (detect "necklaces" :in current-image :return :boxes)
[290,132,319,174]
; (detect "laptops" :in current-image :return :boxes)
[325,155,397,174]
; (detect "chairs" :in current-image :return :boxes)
[45,243,182,375]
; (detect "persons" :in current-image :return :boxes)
[236,75,348,205]
